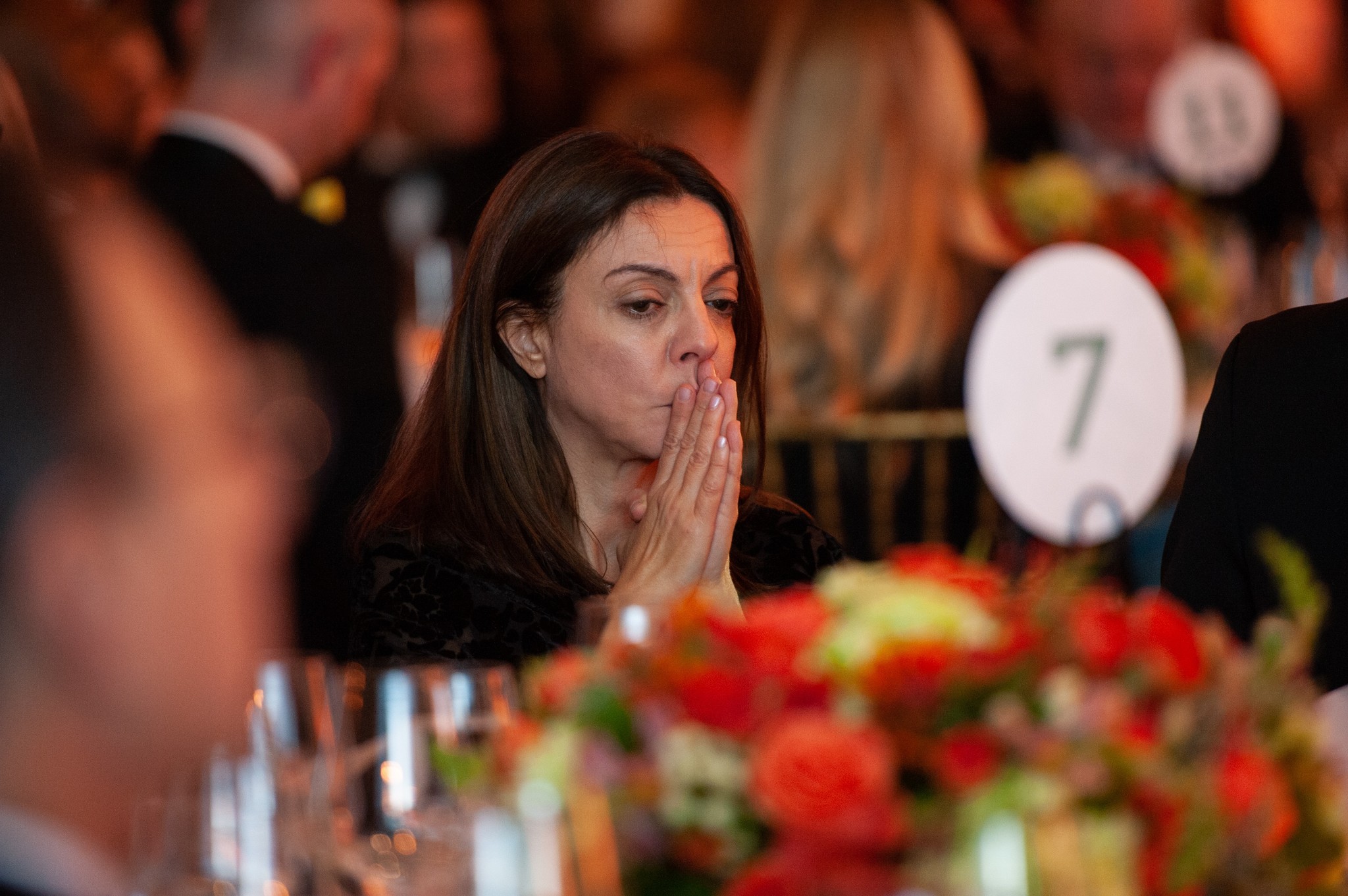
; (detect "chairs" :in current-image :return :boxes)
[757,413,1031,565]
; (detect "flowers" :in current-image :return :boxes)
[414,507,1348,896]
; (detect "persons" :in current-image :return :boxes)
[3,0,1348,749]
[3,151,298,896]
[296,126,844,668]
